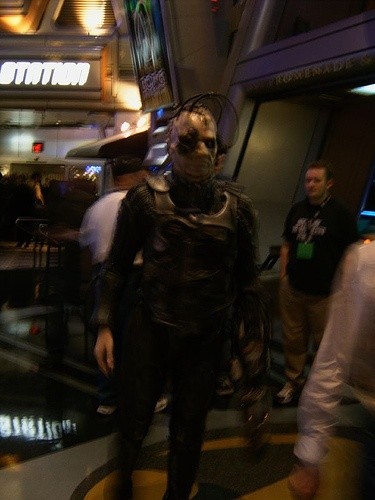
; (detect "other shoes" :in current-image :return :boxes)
[215,375,234,396]
[154,397,169,413]
[96,405,117,414]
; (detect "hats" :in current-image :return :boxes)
[111,156,146,178]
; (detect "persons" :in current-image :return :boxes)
[77,162,168,416]
[0,172,54,249]
[276,161,349,406]
[96,106,267,500]
[285,240,375,500]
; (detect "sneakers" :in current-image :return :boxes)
[275,380,301,404]
